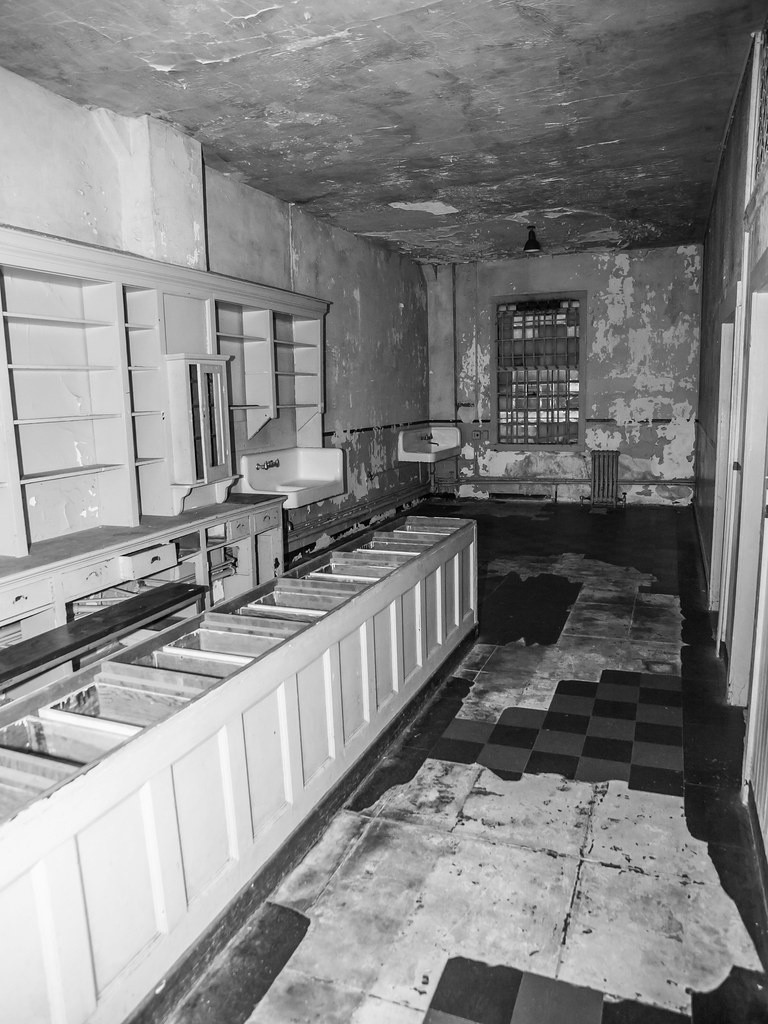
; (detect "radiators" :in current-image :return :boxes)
[590,449,620,505]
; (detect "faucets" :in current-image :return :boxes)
[256,461,269,470]
[428,439,439,446]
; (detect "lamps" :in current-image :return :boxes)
[521,224,542,253]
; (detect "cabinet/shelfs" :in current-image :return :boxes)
[0,493,288,650]
[0,264,178,557]
[214,297,324,448]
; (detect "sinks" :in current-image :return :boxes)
[242,485,309,510]
[398,445,461,462]
